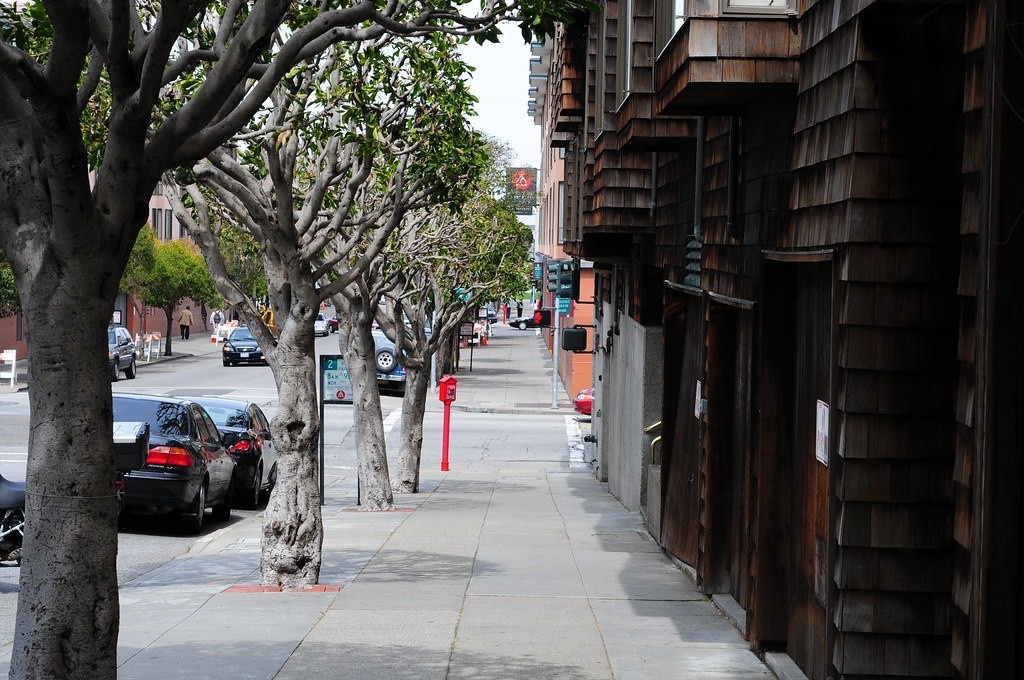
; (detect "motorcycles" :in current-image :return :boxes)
[0,421,149,568]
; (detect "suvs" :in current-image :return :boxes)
[370,330,405,397]
[107,325,137,382]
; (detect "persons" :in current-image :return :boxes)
[260,303,278,336]
[209,307,225,331]
[176,306,194,340]
[506,298,524,319]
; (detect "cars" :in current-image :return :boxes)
[171,396,277,510]
[328,315,339,334]
[371,313,432,340]
[508,314,537,331]
[573,388,592,415]
[222,325,268,367]
[487,304,498,325]
[314,313,330,337]
[110,392,239,536]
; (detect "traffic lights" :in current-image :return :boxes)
[533,309,551,326]
[559,256,581,302]
[546,264,559,296]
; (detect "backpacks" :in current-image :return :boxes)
[214,310,221,324]
